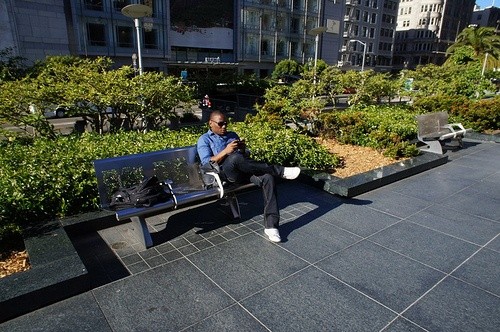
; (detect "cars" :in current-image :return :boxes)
[43,97,119,118]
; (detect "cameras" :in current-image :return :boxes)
[237,141,246,149]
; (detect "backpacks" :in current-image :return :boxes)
[109,176,169,207]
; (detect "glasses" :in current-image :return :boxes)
[212,120,227,126]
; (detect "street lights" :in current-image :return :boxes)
[349,39,367,72]
[307,26,329,114]
[121,3,153,135]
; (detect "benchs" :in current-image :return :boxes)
[92,144,258,248]
[417,111,472,154]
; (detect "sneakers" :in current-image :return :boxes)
[282,167,301,179]
[264,228,281,242]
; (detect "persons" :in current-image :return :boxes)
[197,111,301,243]
